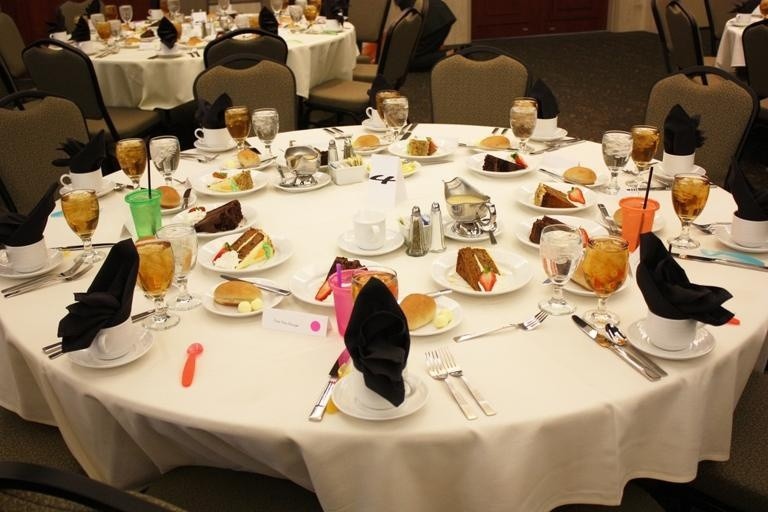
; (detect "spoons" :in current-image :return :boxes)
[605,322,667,377]
[181,342,203,387]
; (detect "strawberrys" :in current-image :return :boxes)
[510,153,527,167]
[188,206,205,212]
[478,265,495,291]
[211,242,231,263]
[567,187,586,204]
[315,282,333,301]
[426,137,435,155]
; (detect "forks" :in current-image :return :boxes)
[454,310,549,343]
[323,128,352,139]
[539,169,605,190]
[4,265,93,298]
[1,259,84,294]
[181,153,219,163]
[423,350,479,420]
[438,346,496,416]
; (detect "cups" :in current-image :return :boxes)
[731,211,768,247]
[646,308,697,351]
[284,146,321,186]
[194,128,230,146]
[355,217,386,250]
[97,317,131,360]
[618,197,660,252]
[50,31,68,40]
[156,223,202,310]
[662,151,695,177]
[60,167,103,192]
[441,177,497,237]
[327,270,361,335]
[0,236,47,273]
[535,117,557,136]
[352,366,416,410]
[124,190,163,239]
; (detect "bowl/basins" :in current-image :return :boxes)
[400,224,432,246]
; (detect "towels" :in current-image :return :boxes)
[0,207,45,247]
[83,1,101,19]
[68,16,90,42]
[258,7,278,36]
[51,129,106,173]
[319,0,350,26]
[57,238,140,354]
[46,14,66,33]
[157,16,177,50]
[527,78,560,118]
[194,92,233,129]
[730,0,761,13]
[635,232,732,329]
[664,104,706,156]
[728,156,768,221]
[344,277,411,407]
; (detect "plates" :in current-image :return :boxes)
[288,259,381,307]
[714,224,768,254]
[626,315,716,360]
[468,153,535,178]
[397,296,463,337]
[0,253,64,278]
[531,128,568,141]
[255,155,274,168]
[444,216,504,243]
[194,140,238,152]
[585,177,609,187]
[388,140,451,158]
[336,232,404,256]
[431,248,534,297]
[197,234,293,273]
[190,168,270,197]
[562,274,629,297]
[649,162,706,181]
[273,172,331,192]
[355,149,385,156]
[59,183,114,198]
[160,191,197,215]
[202,277,284,318]
[330,372,431,421]
[171,203,254,236]
[514,215,609,248]
[518,182,599,214]
[366,160,422,177]
[68,330,155,369]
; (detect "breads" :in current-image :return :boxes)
[564,165,598,186]
[123,36,142,47]
[612,211,623,225]
[153,185,180,207]
[351,133,380,149]
[186,35,203,46]
[237,147,260,167]
[398,292,437,330]
[212,280,263,306]
[479,136,511,150]
[122,21,134,31]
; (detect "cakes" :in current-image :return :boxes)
[206,167,255,194]
[534,180,578,210]
[456,245,503,294]
[210,225,276,273]
[183,199,243,231]
[139,28,156,39]
[529,215,571,245]
[405,133,438,157]
[481,153,525,173]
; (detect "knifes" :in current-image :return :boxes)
[400,123,417,140]
[309,349,351,422]
[43,310,155,354]
[672,252,768,272]
[571,314,660,382]
[220,274,291,296]
[627,184,716,191]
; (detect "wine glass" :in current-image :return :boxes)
[602,130,632,195]
[538,224,583,316]
[60,188,106,264]
[289,6,303,22]
[632,125,660,173]
[224,106,252,150]
[270,1,283,19]
[510,97,537,154]
[253,108,278,156]
[97,22,111,43]
[303,6,318,24]
[149,136,180,186]
[376,90,401,141]
[119,5,132,24]
[90,13,104,29]
[669,172,710,247]
[167,0,179,15]
[116,138,148,190]
[159,0,169,12]
[352,266,399,304]
[134,238,180,331]
[583,235,630,329]
[382,97,408,141]
[104,5,118,20]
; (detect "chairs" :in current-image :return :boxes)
[642,66,759,188]
[428,47,530,129]
[349,1,430,80]
[651,1,736,72]
[21,39,158,151]
[202,27,288,69]
[306,8,423,128]
[1,12,36,81]
[1,91,92,223]
[191,53,295,135]
